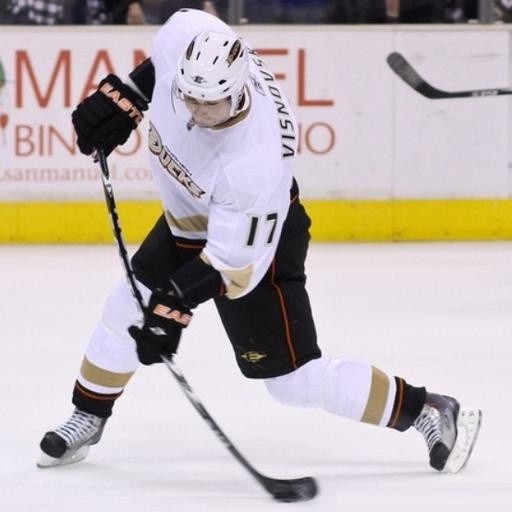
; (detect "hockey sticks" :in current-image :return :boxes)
[96,148,317,502]
[387,53,512,99]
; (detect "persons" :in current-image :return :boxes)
[0,0,512,25]
[40,4,460,471]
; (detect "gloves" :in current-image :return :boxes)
[126,286,195,365]
[67,74,151,162]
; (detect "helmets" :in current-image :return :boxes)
[171,28,250,119]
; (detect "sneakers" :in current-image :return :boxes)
[40,407,110,459]
[410,391,461,471]
